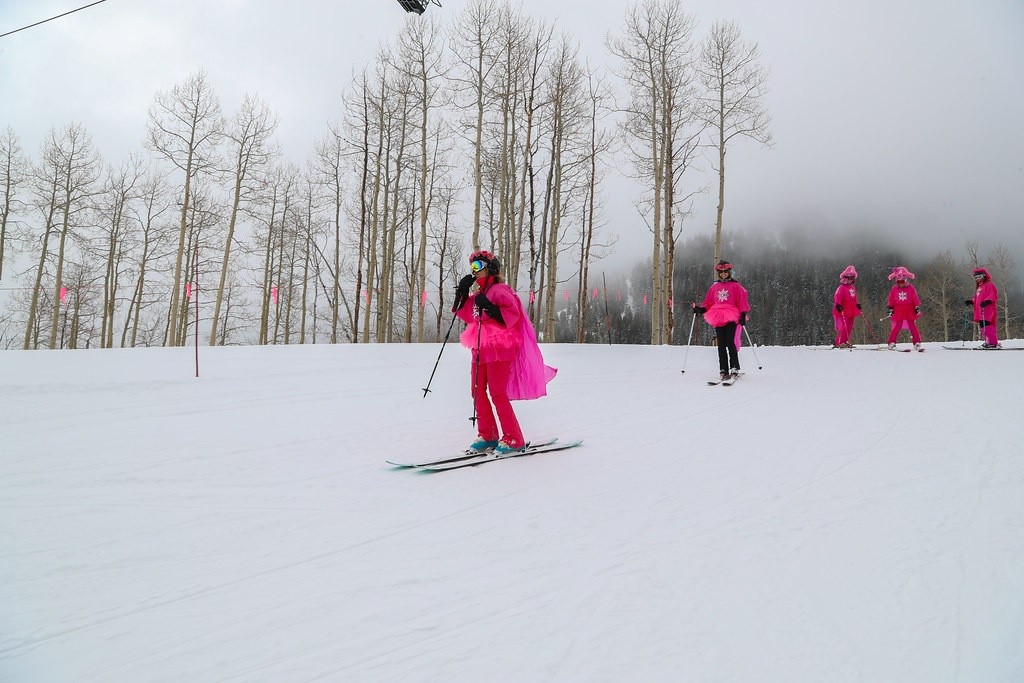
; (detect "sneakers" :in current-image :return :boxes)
[470,434,499,454]
[493,440,526,455]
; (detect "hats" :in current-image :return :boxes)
[840,266,857,278]
[715,261,732,271]
[469,251,500,275]
[973,270,986,280]
[889,267,914,281]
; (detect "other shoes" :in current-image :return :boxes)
[838,342,852,348]
[915,343,920,350]
[981,342,998,348]
[731,368,738,379]
[720,371,729,380]
[888,343,896,350]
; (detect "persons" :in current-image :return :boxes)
[887,267,921,350]
[964,268,998,348]
[452,250,557,456]
[833,266,862,348]
[693,262,751,379]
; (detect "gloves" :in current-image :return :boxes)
[887,307,894,316]
[965,299,974,306]
[474,293,492,311]
[739,315,746,326]
[458,273,477,294]
[915,307,919,314]
[692,305,702,315]
[980,301,990,308]
[836,304,843,312]
[857,304,862,309]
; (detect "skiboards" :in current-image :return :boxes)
[882,345,925,352]
[809,345,881,351]
[941,344,1024,351]
[386,436,584,471]
[707,372,745,386]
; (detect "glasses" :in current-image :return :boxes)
[471,259,486,273]
[975,275,983,280]
[719,269,728,273]
